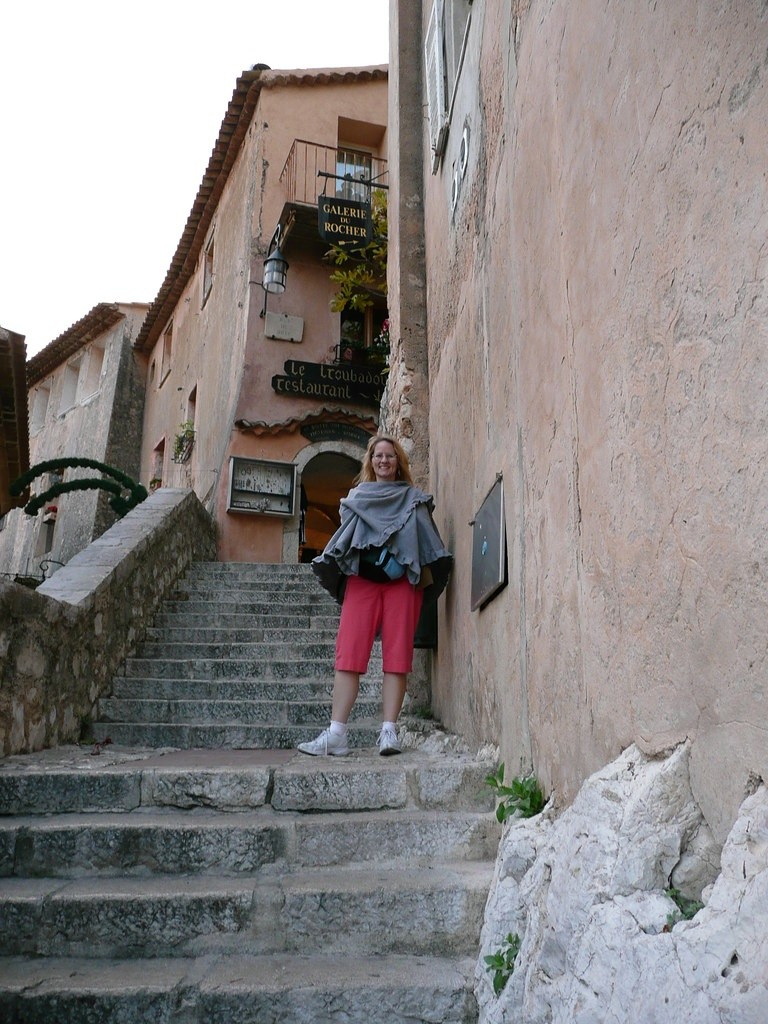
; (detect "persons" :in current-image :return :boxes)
[295,432,456,755]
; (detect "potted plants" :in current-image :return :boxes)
[150,478,162,491]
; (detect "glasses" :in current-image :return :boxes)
[372,453,397,461]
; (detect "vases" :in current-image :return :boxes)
[42,513,56,524]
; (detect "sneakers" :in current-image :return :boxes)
[297,730,348,756]
[376,729,401,755]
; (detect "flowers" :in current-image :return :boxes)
[44,505,57,514]
[339,319,390,373]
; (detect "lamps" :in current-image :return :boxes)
[260,224,290,316]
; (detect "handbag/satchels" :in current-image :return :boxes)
[373,549,406,578]
